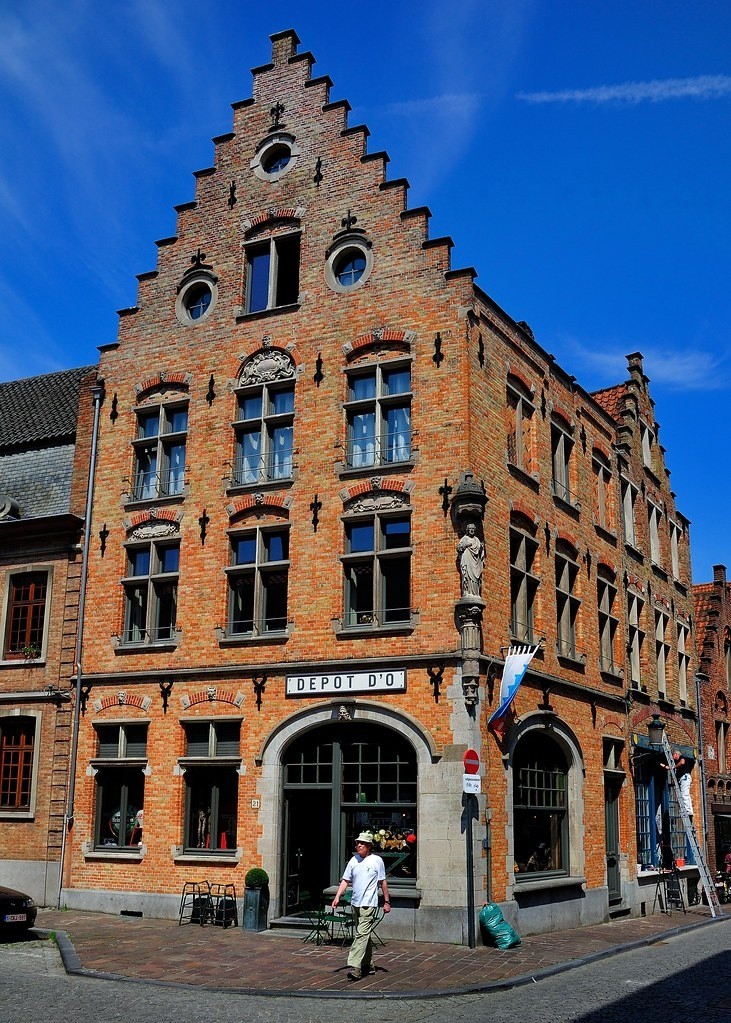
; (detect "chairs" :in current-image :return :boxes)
[337,890,390,950]
[301,899,335,946]
[319,896,352,950]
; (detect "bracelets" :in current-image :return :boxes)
[385,901,390,904]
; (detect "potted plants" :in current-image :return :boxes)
[5,646,42,660]
[242,868,270,932]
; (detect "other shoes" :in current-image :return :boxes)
[347,967,362,980]
[361,965,375,974]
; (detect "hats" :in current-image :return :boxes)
[355,833,374,847]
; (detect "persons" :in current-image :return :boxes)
[659,751,693,815]
[332,833,391,979]
[457,524,485,599]
[725,847,731,879]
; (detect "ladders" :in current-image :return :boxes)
[662,731,724,918]
[651,858,687,916]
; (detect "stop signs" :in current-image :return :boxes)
[463,749,479,775]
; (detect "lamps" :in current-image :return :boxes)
[631,714,666,760]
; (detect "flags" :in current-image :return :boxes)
[487,645,539,723]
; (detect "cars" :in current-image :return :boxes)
[0,886,37,938]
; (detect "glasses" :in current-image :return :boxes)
[356,840,370,846]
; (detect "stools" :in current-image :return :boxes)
[178,878,239,930]
